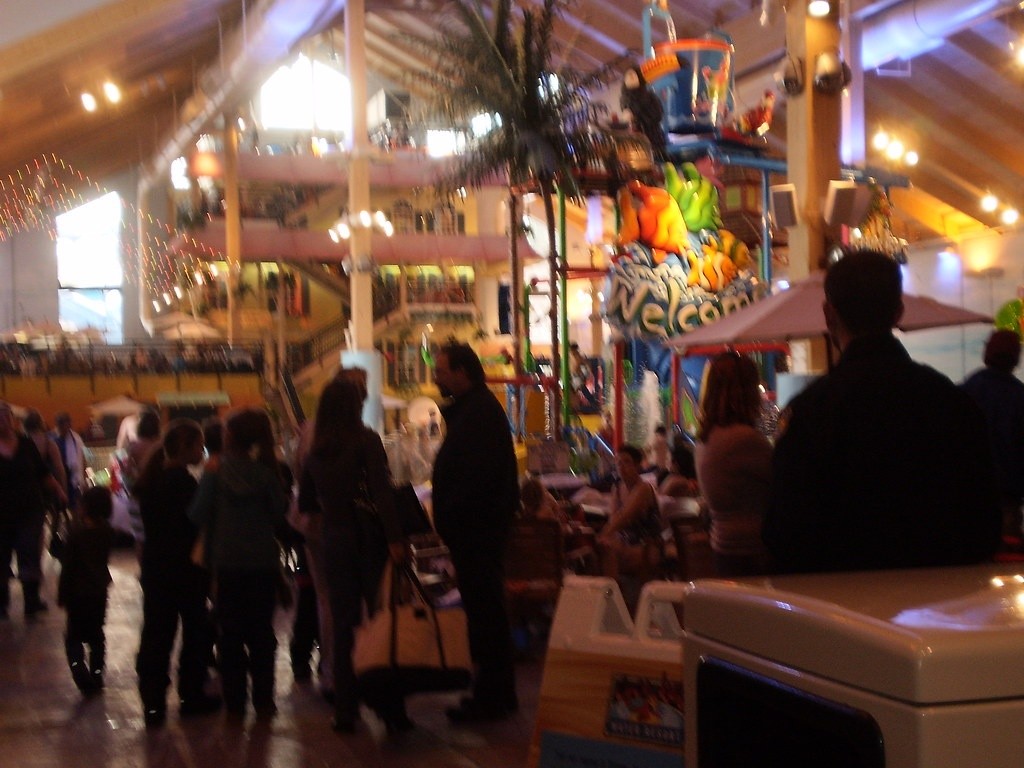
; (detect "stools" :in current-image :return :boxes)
[504,579,556,661]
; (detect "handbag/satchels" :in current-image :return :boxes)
[351,556,472,696]
[190,525,208,573]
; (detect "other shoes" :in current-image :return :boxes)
[90,661,106,688]
[27,600,49,614]
[389,716,412,736]
[181,693,222,717]
[144,698,166,724]
[72,660,96,699]
[330,715,355,733]
[446,690,519,722]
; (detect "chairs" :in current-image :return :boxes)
[388,543,465,674]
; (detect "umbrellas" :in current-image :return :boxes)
[668,272,996,376]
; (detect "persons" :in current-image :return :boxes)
[700,350,774,576]
[120,404,294,726]
[521,480,559,519]
[568,340,595,406]
[432,344,520,721]
[381,123,409,147]
[764,251,1024,572]
[651,428,669,472]
[290,368,416,736]
[596,446,665,590]
[0,401,135,691]
